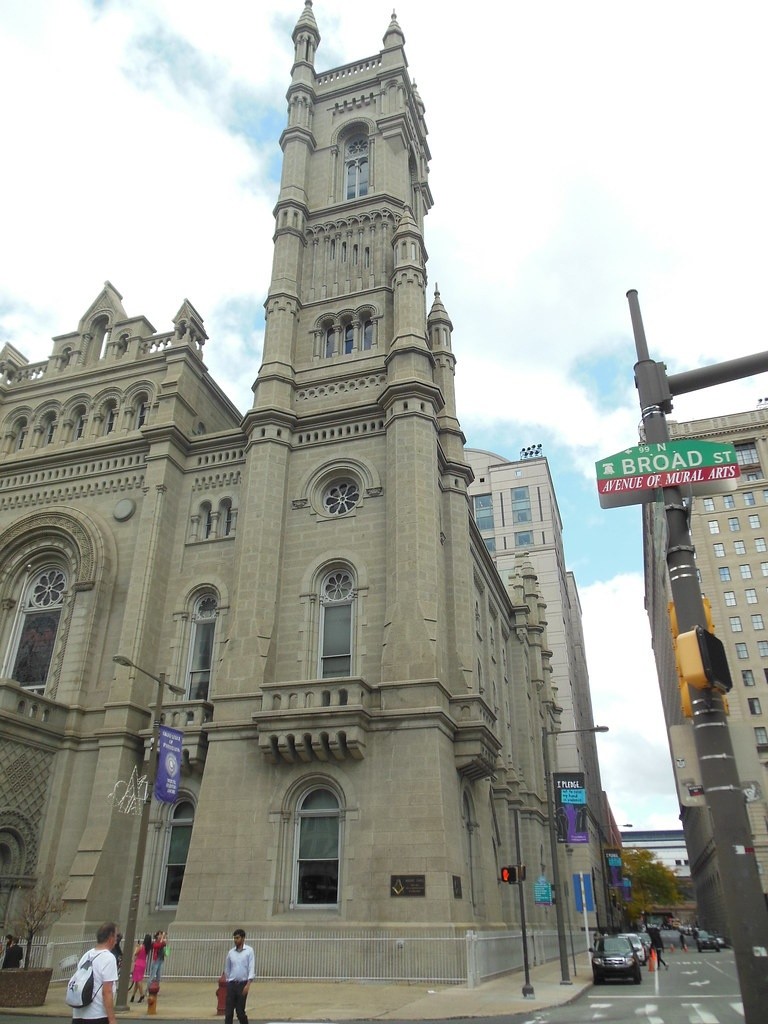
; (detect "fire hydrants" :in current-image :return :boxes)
[146,977,160,1016]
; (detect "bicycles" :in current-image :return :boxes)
[112,957,135,992]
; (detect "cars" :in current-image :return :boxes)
[616,932,646,966]
[641,916,727,948]
[632,932,653,959]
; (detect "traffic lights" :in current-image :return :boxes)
[675,624,733,695]
[501,866,519,883]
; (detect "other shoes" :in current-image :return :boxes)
[664,963,669,970]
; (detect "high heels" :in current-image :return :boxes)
[130,995,134,1002]
[138,995,145,1003]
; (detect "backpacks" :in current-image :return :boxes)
[65,951,105,1008]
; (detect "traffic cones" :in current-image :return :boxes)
[650,948,657,962]
[647,956,658,972]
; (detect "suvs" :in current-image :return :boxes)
[588,935,642,985]
[694,928,720,953]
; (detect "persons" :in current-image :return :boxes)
[224,928,255,1024]
[680,932,687,952]
[71,922,119,1024]
[110,931,166,1003]
[649,933,669,970]
[2,934,23,969]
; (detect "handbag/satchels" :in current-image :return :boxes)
[161,945,170,957]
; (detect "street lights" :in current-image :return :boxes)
[541,725,611,985]
[596,822,633,935]
[111,654,188,1011]
